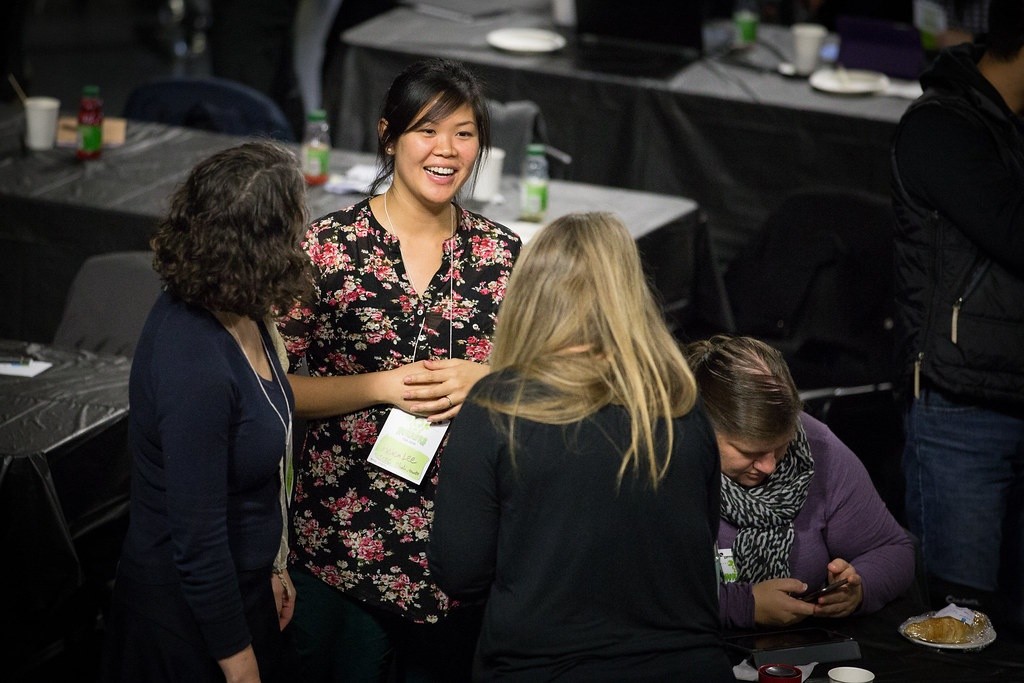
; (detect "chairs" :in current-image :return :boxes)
[679,191,897,417]
[47,250,161,359]
[122,74,293,145]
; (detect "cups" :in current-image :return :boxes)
[24,96,60,152]
[792,23,828,76]
[731,8,758,50]
[828,666,875,683]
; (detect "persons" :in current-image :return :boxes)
[881,0,1024,595]
[686,334,915,624]
[128,142,314,683]
[428,211,723,683]
[271,56,523,683]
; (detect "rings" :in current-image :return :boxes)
[445,396,452,407]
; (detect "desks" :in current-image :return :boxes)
[343,2,919,272]
[0,340,130,628]
[716,610,1024,683]
[0,111,698,315]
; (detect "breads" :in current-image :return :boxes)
[905,608,991,645]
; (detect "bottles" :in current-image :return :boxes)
[76,86,105,158]
[299,109,332,186]
[516,144,552,222]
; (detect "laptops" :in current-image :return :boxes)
[574,0,736,61]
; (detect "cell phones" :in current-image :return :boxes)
[799,578,849,603]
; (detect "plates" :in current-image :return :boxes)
[486,26,567,54]
[900,613,997,649]
[809,67,890,95]
